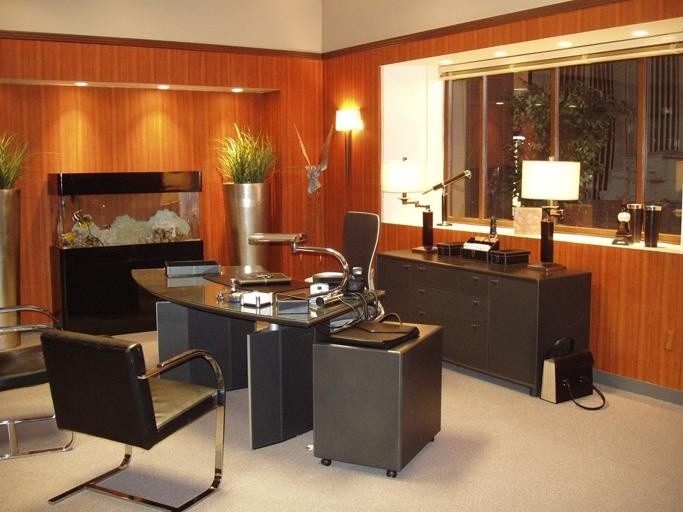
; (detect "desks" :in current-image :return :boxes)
[129,262,386,451]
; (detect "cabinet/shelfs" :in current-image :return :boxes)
[374,246,591,397]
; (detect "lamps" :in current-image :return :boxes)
[382,157,471,254]
[247,225,366,309]
[333,108,364,175]
[521,157,582,272]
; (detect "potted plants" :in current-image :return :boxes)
[0,132,36,348]
[215,119,282,272]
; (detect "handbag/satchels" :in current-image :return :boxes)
[538,336,605,410]
[327,311,419,350]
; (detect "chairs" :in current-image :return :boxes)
[41,327,226,512]
[0,306,73,461]
[287,210,380,293]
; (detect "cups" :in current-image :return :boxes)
[626,204,662,248]
